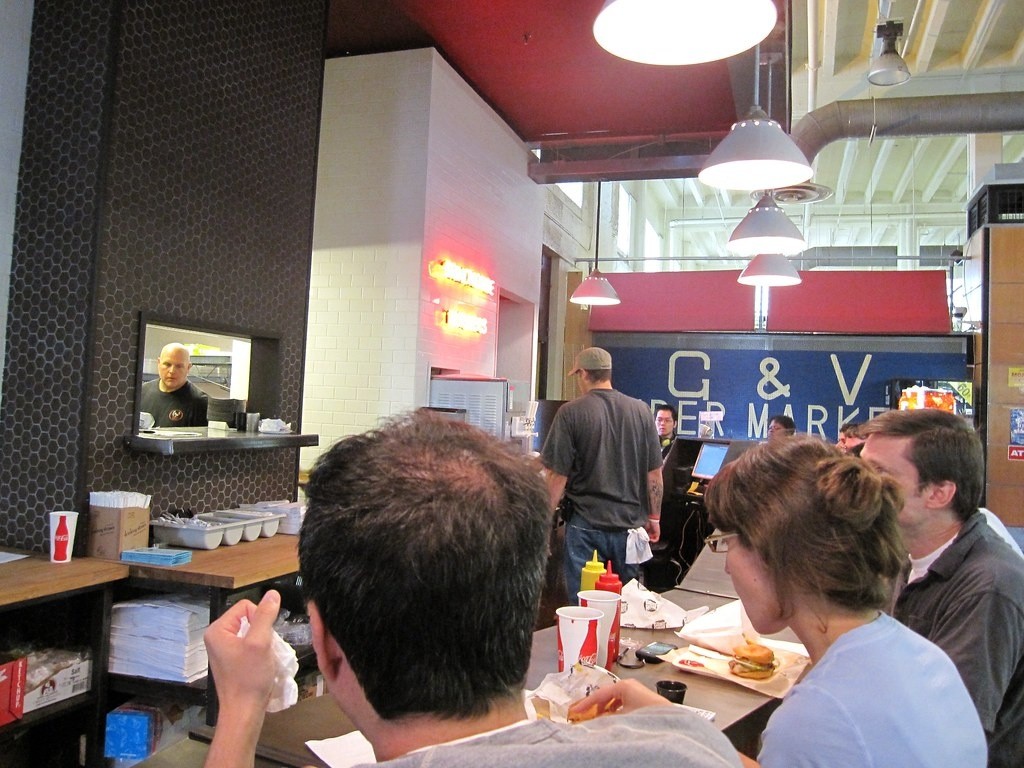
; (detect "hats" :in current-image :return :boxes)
[567,346,612,376]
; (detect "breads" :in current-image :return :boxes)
[568,697,619,722]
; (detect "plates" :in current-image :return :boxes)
[259,429,292,434]
[207,396,246,428]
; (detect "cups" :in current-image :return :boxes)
[556,605,605,673]
[512,401,539,435]
[655,680,688,705]
[235,411,260,432]
[577,590,622,673]
[49,511,79,564]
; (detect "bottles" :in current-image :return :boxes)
[505,421,510,440]
[595,560,622,662]
[580,550,608,591]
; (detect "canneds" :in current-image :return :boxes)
[898,388,956,415]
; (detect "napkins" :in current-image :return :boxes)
[305,730,376,768]
[107,591,210,684]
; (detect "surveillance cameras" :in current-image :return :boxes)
[954,307,968,317]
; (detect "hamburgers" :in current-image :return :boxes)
[728,643,777,680]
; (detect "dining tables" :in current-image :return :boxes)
[128,544,809,768]
[1,534,315,768]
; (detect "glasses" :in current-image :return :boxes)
[704,531,744,553]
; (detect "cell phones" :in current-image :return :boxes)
[635,642,678,664]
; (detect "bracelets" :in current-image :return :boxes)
[646,514,661,523]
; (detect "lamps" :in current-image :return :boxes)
[729,50,806,254]
[569,181,621,306]
[866,20,911,87]
[697,43,814,191]
[736,189,801,287]
[592,0,778,65]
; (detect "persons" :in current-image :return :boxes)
[141,343,210,427]
[653,405,678,460]
[767,414,797,441]
[569,433,989,768]
[538,346,665,607]
[834,408,1024,768]
[203,406,744,768]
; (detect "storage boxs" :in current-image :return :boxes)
[104,697,190,759]
[88,505,150,562]
[0,648,92,725]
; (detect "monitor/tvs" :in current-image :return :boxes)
[691,442,729,480]
